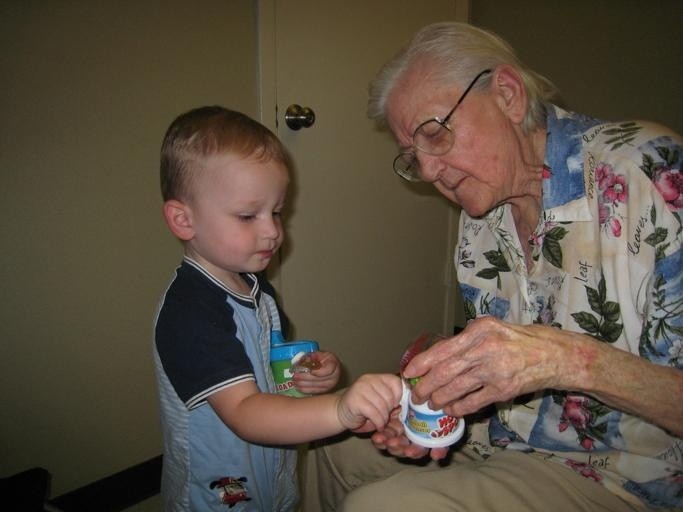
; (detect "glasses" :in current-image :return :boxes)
[392,68,494,185]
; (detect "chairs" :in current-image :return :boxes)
[0,466,51,512]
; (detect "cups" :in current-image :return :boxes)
[400,379,466,448]
[270,330,319,398]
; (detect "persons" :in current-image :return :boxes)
[147,105,406,512]
[290,20,681,512]
